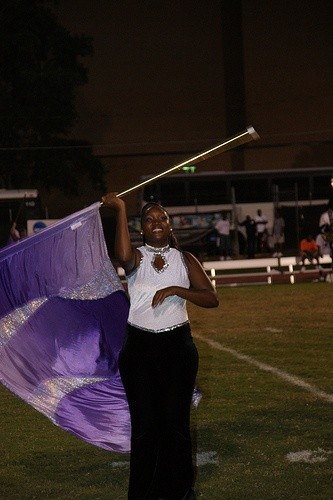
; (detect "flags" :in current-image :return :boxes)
[0,203,203,452]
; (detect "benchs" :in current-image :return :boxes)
[202,255,333,288]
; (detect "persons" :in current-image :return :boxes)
[101,190,221,500]
[7,218,22,244]
[215,198,333,272]
